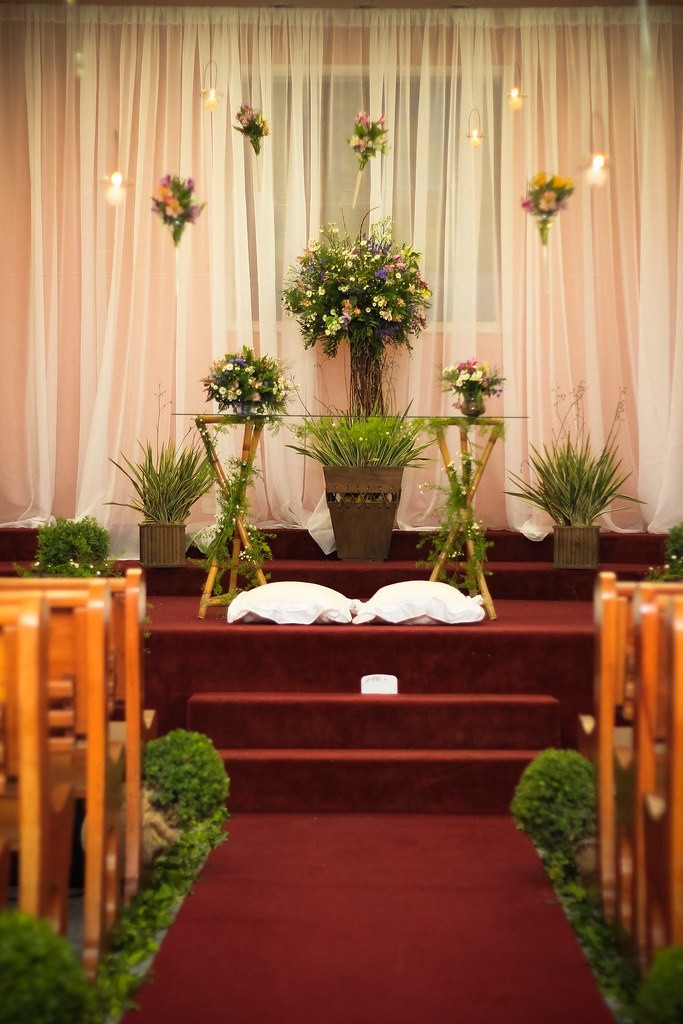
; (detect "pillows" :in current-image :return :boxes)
[224,580,361,628]
[353,579,486,626]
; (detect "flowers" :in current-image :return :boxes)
[277,211,433,418]
[438,358,504,408]
[198,345,289,413]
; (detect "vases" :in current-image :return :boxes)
[457,388,487,417]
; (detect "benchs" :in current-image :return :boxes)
[2,568,683,991]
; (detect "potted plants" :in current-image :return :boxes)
[100,426,218,568]
[289,397,436,565]
[504,432,648,576]
